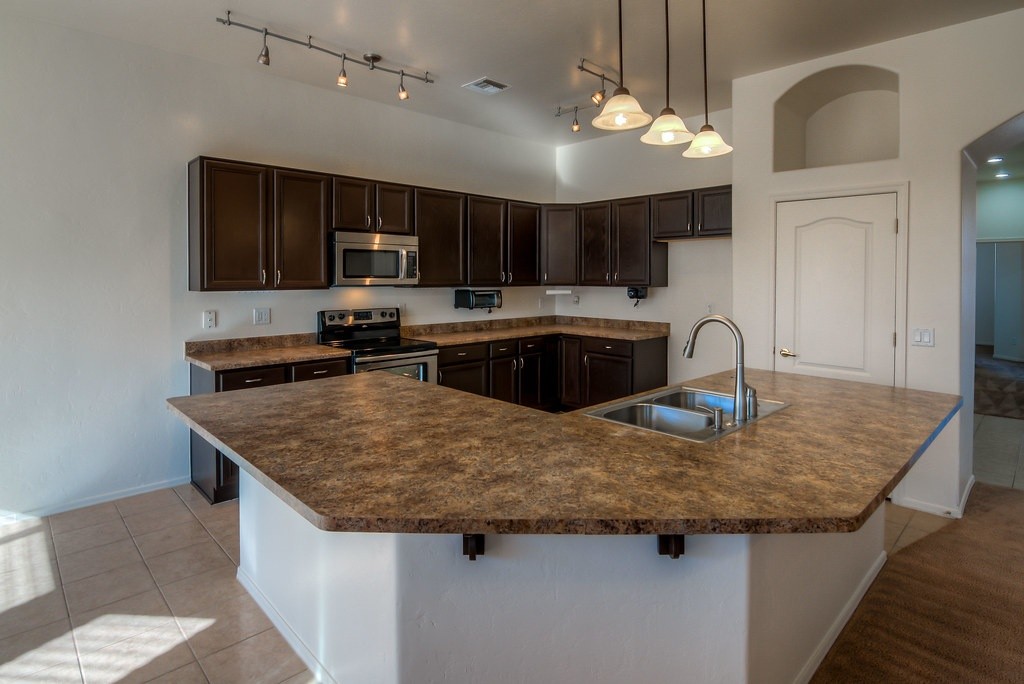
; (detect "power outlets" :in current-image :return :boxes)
[253,308,271,325]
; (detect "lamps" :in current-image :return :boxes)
[571,107,580,132]
[640,0,696,146]
[337,53,348,87]
[591,0,652,131]
[256,28,269,65]
[592,89,606,105]
[398,70,409,100]
[682,0,732,159]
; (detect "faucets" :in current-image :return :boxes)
[683,314,748,421]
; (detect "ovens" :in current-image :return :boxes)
[351,349,439,385]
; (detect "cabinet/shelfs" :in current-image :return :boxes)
[187,155,732,289]
[188,332,668,507]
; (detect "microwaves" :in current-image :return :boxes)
[332,231,420,284]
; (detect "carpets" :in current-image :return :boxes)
[975,344,1024,420]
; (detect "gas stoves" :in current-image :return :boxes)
[317,308,437,356]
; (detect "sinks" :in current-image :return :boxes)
[605,404,713,433]
[653,392,759,415]
